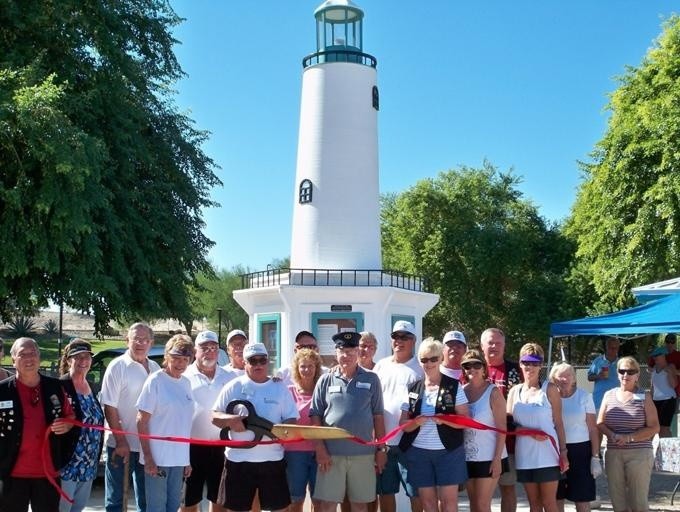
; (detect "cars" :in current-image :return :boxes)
[87,336,233,399]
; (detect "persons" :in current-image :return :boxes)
[134,333,196,511]
[597,357,660,511]
[280,348,339,512]
[210,341,301,511]
[509,343,571,512]
[436,331,475,511]
[220,328,263,512]
[628,333,680,437]
[0,338,14,381]
[550,363,602,511]
[58,338,105,511]
[275,330,332,385]
[308,332,389,511]
[339,331,379,511]
[399,336,469,511]
[0,336,78,511]
[100,323,162,511]
[460,350,507,511]
[177,330,283,512]
[588,336,622,455]
[459,328,543,512]
[372,319,427,512]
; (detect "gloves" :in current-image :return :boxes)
[590,456,602,480]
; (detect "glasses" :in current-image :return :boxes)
[156,469,168,479]
[248,357,268,365]
[521,362,542,367]
[30,386,40,407]
[420,356,440,364]
[196,345,219,354]
[391,332,415,341]
[296,345,318,351]
[129,335,154,345]
[618,369,639,375]
[463,362,484,370]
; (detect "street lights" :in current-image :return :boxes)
[55,292,64,361]
[215,306,224,349]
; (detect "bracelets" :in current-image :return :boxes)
[559,447,568,452]
[592,453,600,457]
[613,432,616,442]
[627,432,630,443]
[630,432,634,442]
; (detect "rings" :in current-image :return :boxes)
[319,463,322,467]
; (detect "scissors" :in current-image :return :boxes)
[219,399,356,449]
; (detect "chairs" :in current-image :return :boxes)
[650,437,680,507]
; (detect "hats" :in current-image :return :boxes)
[519,352,543,363]
[226,329,248,346]
[243,342,269,362]
[332,330,362,349]
[441,329,467,347]
[648,345,669,357]
[393,320,417,337]
[195,330,220,347]
[168,346,194,357]
[66,343,94,359]
[459,355,486,367]
[295,330,317,343]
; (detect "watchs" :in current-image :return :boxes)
[377,446,387,452]
